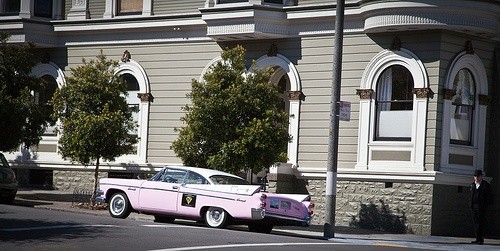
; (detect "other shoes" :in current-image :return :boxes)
[471,240,484,244]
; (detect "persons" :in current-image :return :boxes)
[467,169,493,245]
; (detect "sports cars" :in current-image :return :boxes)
[92,164,315,234]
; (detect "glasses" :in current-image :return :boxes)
[474,175,480,177]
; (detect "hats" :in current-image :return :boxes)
[472,169,483,175]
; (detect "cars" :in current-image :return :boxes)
[0,153,18,204]
